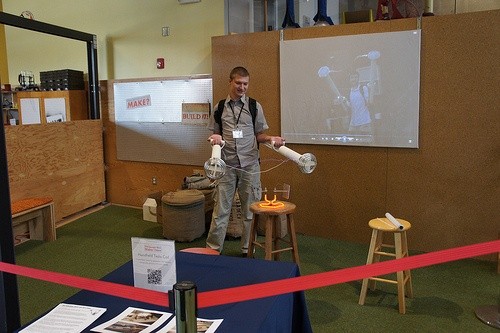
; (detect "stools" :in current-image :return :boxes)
[250,199,301,265]
[357,218,412,315]
[182,248,221,255]
[162,187,206,244]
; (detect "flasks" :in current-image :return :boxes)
[172,280,198,333]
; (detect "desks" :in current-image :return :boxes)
[15,251,315,333]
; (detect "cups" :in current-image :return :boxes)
[9,119,16,126]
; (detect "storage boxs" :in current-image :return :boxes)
[39,69,84,90]
[143,197,163,223]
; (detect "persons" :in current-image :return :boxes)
[342,71,372,135]
[205,66,286,257]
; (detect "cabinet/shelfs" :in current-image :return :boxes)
[17,90,87,128]
[0,90,17,124]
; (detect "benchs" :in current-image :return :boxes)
[12,197,56,241]
